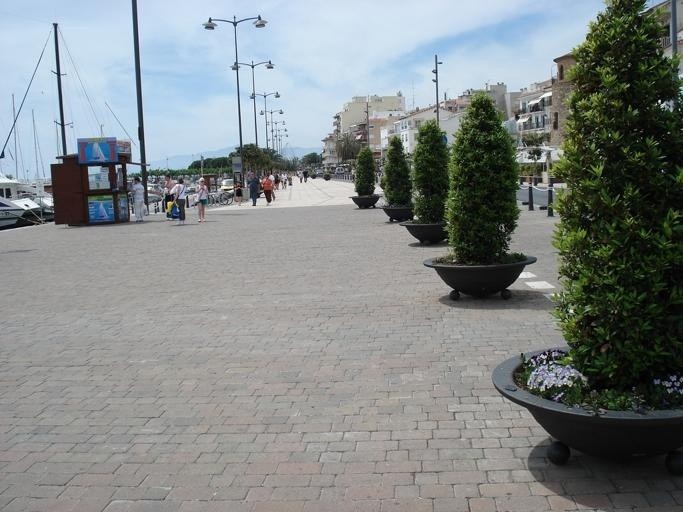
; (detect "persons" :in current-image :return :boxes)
[169,176,187,221]
[161,175,176,211]
[233,168,308,207]
[126,177,144,222]
[195,178,208,222]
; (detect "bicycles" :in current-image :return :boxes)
[205,190,233,207]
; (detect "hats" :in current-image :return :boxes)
[178,176,183,184]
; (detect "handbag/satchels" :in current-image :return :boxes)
[166,202,180,218]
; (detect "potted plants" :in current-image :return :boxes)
[492,2,682,468]
[352,95,538,300]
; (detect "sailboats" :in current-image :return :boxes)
[0,94,53,230]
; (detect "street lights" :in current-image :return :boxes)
[204,14,266,186]
[230,60,274,146]
[249,90,279,151]
[364,103,374,149]
[431,55,442,127]
[259,108,288,155]
